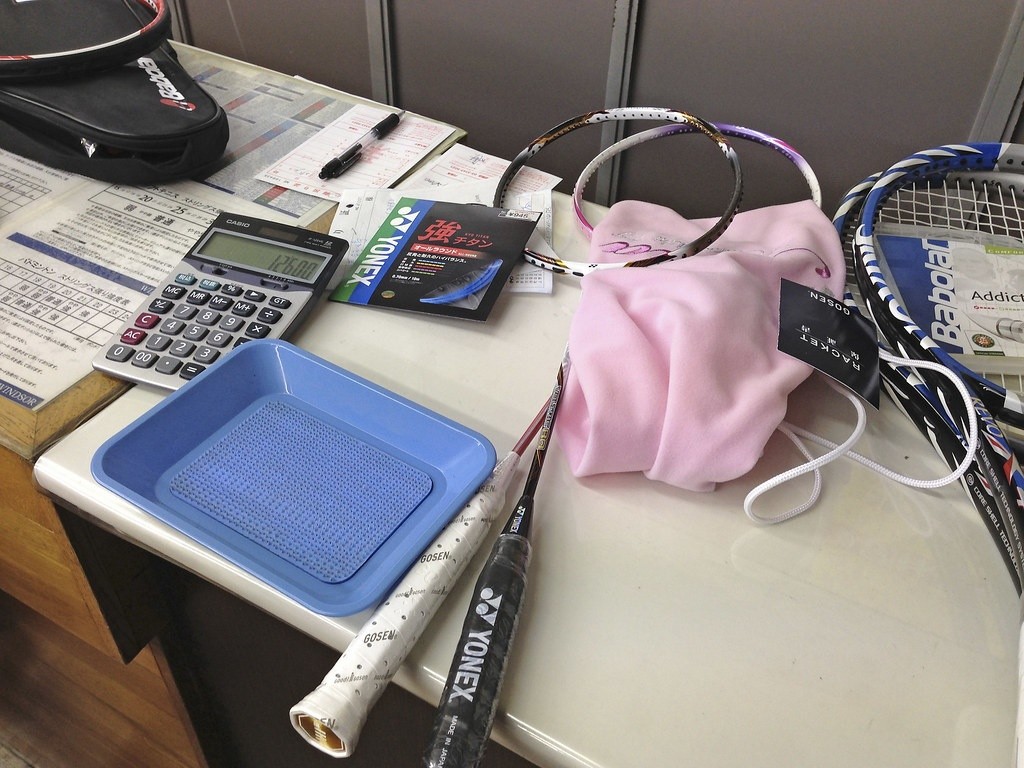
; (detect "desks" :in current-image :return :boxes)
[0,37,1024,768]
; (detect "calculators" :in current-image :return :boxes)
[91,212,350,395]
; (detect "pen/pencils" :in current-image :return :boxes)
[319,108,406,182]
